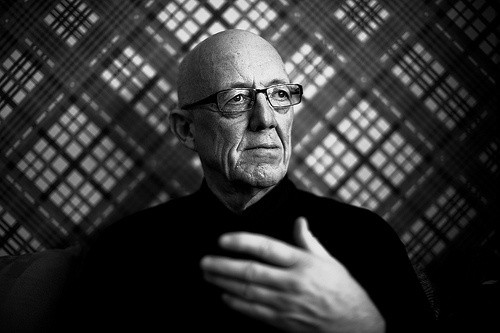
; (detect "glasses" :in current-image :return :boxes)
[179,84,303,114]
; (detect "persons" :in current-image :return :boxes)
[48,29,443,333]
